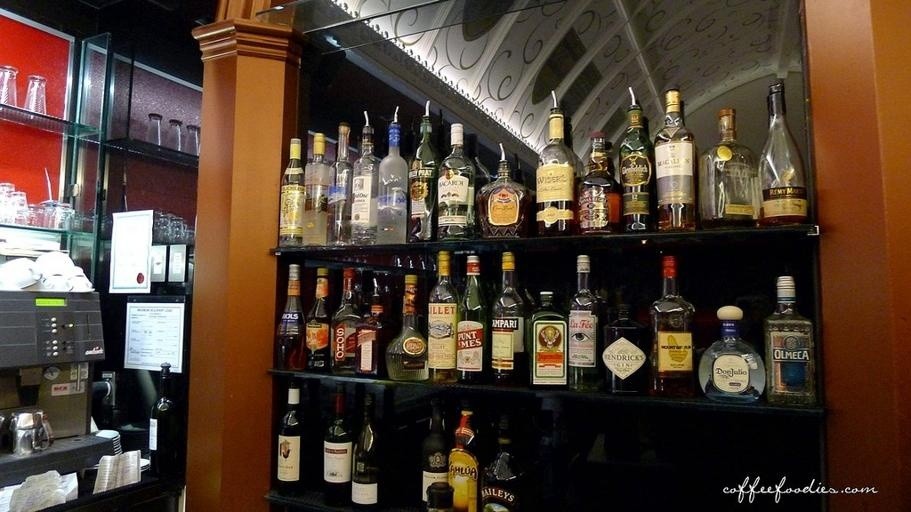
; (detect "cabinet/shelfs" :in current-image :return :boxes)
[0,7,104,285]
[262,223,831,511]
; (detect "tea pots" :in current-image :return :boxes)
[7,408,52,458]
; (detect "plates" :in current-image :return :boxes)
[92,429,123,469]
[141,458,151,472]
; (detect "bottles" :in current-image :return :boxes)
[148,363,178,478]
[274,383,525,512]
[275,244,819,408]
[277,81,809,250]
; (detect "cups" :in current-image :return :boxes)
[145,113,201,157]
[0,64,47,115]
[152,210,194,245]
[0,182,82,234]
